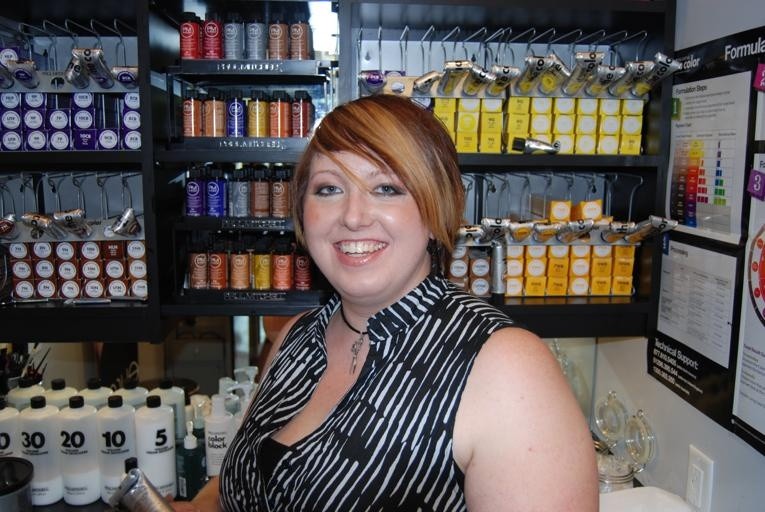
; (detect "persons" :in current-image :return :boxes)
[163,95,600,511]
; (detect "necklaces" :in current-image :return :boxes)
[338,302,369,373]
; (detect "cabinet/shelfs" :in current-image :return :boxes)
[167,58,332,314]
[2,1,182,341]
[161,313,235,399]
[341,2,675,339]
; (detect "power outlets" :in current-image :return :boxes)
[685,443,716,512]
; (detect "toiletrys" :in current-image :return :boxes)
[180,364,262,501]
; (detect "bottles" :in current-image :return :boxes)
[175,10,311,296]
[581,391,657,492]
[0,365,258,508]
